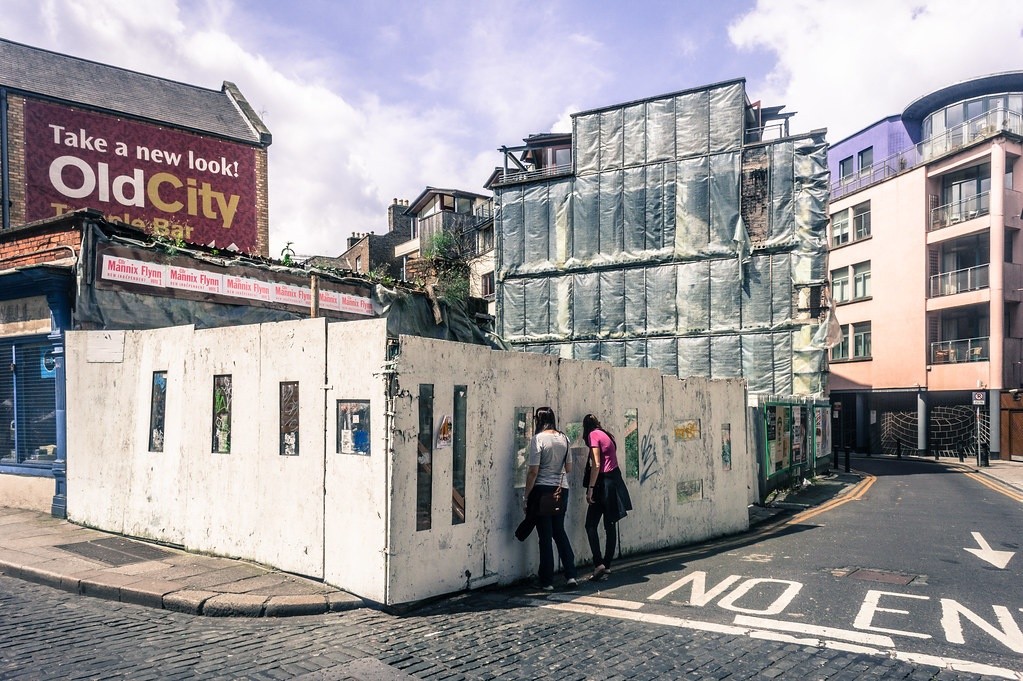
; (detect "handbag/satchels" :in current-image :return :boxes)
[553,473,565,513]
[583,452,592,488]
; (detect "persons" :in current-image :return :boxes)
[521,406,580,590]
[582,414,632,579]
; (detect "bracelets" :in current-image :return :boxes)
[588,485,593,488]
[522,496,527,500]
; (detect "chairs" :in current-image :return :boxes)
[965,347,982,362]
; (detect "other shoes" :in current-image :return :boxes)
[563,578,578,587]
[588,564,611,581]
[531,582,554,591]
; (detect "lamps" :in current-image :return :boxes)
[977,380,982,388]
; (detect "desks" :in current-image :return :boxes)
[951,218,959,224]
[936,350,957,364]
